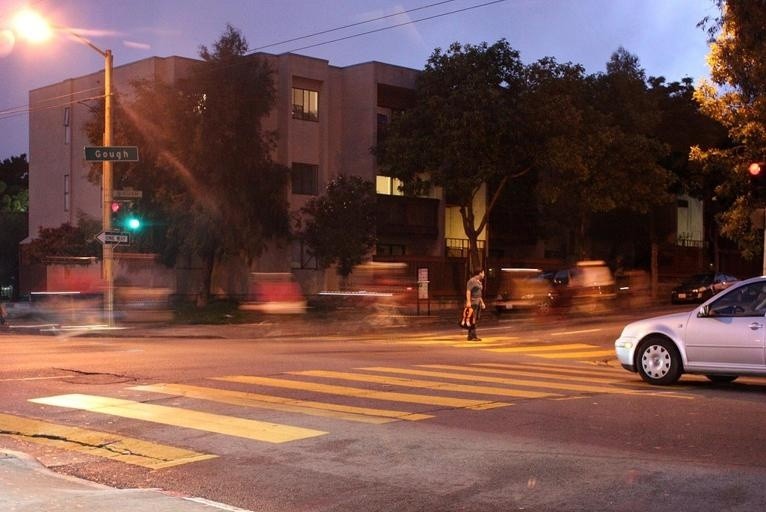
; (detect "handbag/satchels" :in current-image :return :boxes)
[459,305,478,330]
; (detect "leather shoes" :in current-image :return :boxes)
[467,337,479,341]
[476,337,482,341]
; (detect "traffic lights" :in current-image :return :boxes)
[110,202,122,227]
[748,162,765,176]
[123,216,138,232]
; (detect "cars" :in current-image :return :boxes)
[494,265,613,314]
[614,275,766,383]
[672,272,739,303]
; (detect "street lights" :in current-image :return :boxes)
[19,9,115,330]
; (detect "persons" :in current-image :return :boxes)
[466,267,485,341]
[698,266,741,310]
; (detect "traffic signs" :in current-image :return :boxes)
[111,189,142,203]
[95,231,129,246]
